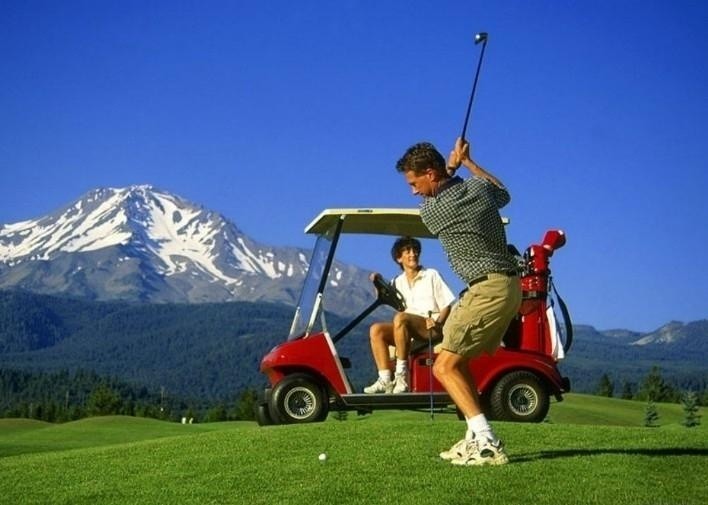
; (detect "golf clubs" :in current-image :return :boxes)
[428,311,434,421]
[461,33,488,143]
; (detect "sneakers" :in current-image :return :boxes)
[450,437,511,468]
[438,429,476,461]
[363,377,393,394]
[392,371,410,393]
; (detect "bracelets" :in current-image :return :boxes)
[447,166,457,172]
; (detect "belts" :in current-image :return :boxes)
[466,269,517,287]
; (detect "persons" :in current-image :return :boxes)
[363,237,456,394]
[395,136,524,467]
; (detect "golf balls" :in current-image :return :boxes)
[318,453,326,460]
